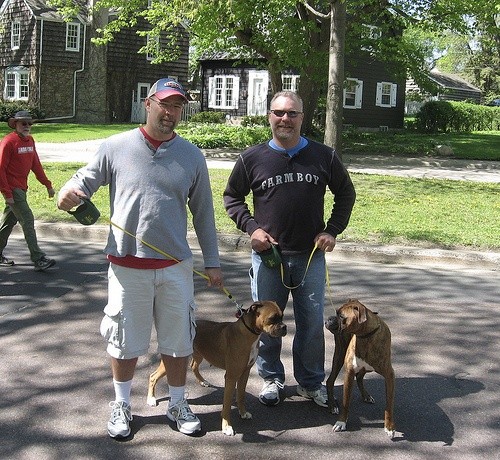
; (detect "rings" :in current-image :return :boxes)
[73,203,77,206]
[322,244,326,249]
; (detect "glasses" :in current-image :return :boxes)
[16,120,34,126]
[269,109,304,119]
[149,97,184,112]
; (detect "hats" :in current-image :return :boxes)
[148,78,189,106]
[8,111,38,129]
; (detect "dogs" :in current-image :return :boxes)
[147,300,287,436]
[326,298,395,441]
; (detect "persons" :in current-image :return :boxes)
[223,90,356,408]
[0,111,55,271]
[57,78,224,437]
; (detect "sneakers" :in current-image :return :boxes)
[166,392,201,436]
[34,256,56,272]
[296,382,336,408]
[258,379,284,406]
[107,400,133,439]
[0,255,14,267]
